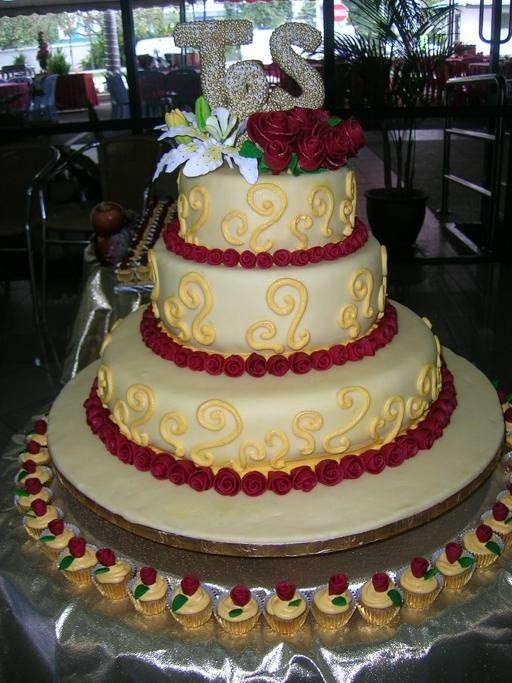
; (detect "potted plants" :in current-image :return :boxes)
[328,2,457,251]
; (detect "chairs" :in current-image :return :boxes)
[0,140,66,386]
[29,135,162,380]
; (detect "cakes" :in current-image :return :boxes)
[83,21,456,498]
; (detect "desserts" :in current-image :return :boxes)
[10,382,511,633]
